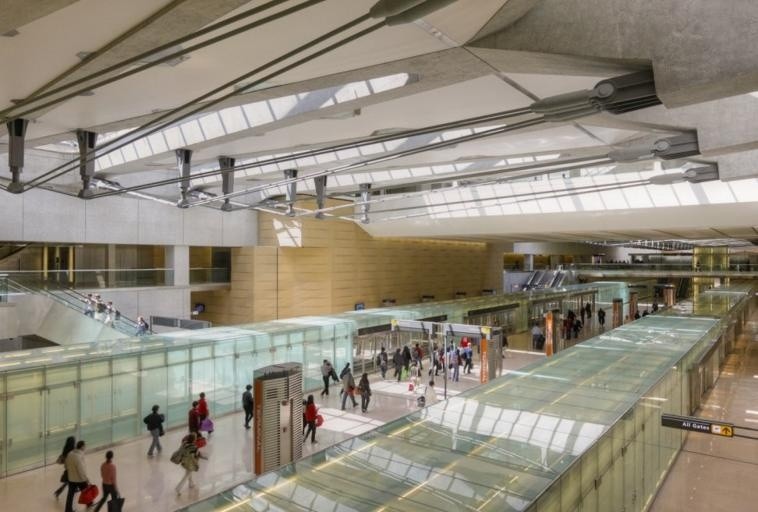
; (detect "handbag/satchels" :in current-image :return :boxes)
[355,387,362,395]
[107,498,124,512]
[201,418,213,431]
[196,439,206,447]
[418,396,426,407]
[171,448,187,464]
[78,484,99,505]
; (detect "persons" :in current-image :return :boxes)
[502,323,545,359]
[53,436,76,500]
[134,316,147,336]
[624,304,658,324]
[93,294,108,320]
[303,341,474,444]
[63,440,96,511]
[103,300,120,328]
[77,293,94,316]
[94,450,119,512]
[141,385,254,498]
[563,302,606,340]
